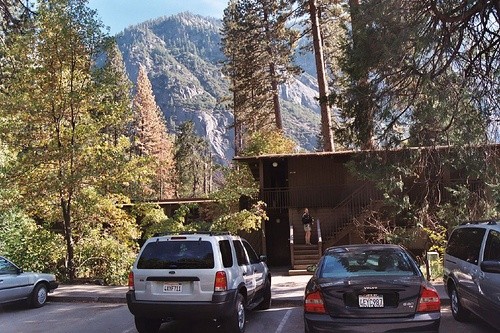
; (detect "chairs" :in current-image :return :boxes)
[375,254,398,271]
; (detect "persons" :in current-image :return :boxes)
[302,208,315,246]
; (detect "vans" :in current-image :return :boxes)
[126,233,271,333]
[439,221,500,333]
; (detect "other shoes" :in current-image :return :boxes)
[306,244,311,246]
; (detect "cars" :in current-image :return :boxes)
[303,244,441,333]
[0,257,58,308]
